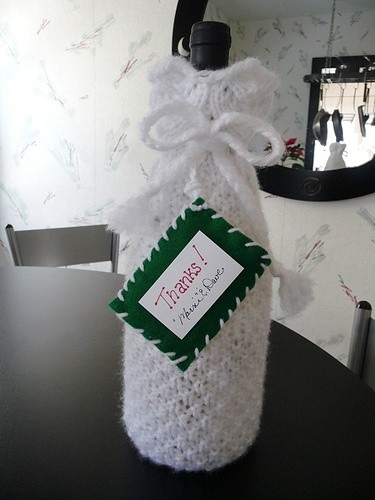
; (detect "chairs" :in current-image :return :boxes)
[5,223,120,273]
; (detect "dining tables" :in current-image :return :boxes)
[0,267,375,500]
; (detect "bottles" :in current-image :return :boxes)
[117,21,270,471]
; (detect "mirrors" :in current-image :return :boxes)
[171,0,374,201]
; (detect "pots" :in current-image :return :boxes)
[312,90,330,147]
[358,84,368,136]
[333,89,344,141]
